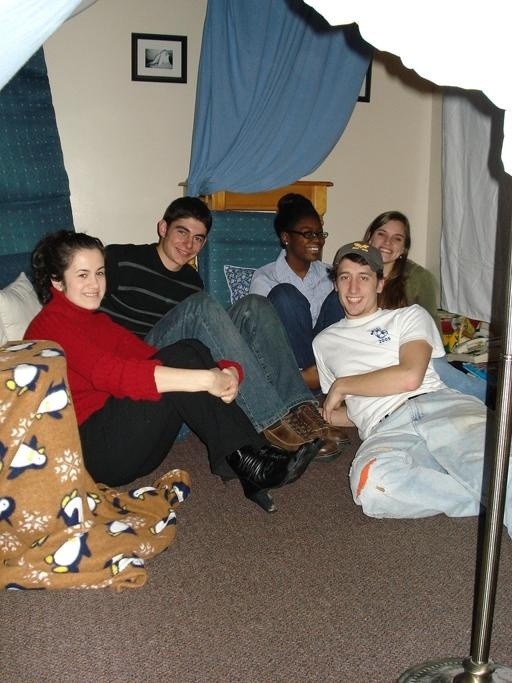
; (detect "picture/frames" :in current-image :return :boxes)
[129,30,189,85]
[352,54,373,104]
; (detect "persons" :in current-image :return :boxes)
[96,195,351,463]
[311,241,512,540]
[250,193,345,399]
[24,228,324,514]
[363,211,441,329]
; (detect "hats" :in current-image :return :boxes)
[333,242,384,271]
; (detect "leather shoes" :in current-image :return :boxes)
[263,401,351,463]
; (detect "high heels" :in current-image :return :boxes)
[226,438,323,513]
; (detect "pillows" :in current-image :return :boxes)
[0,268,46,343]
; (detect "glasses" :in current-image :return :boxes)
[294,230,328,240]
